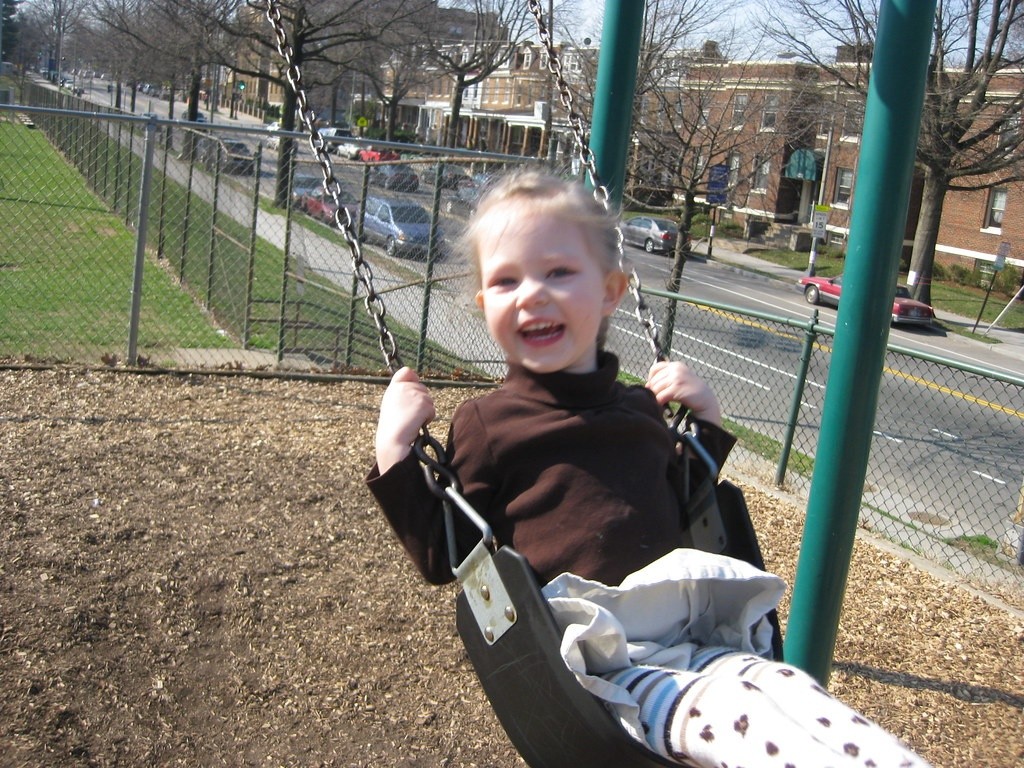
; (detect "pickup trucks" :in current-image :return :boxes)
[358,146,403,165]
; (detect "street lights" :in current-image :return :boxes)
[779,51,844,276]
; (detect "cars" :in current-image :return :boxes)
[301,185,359,226]
[619,216,692,256]
[796,272,934,329]
[445,174,496,219]
[193,137,254,177]
[136,82,170,102]
[181,111,208,122]
[420,161,473,190]
[337,144,365,160]
[367,164,420,192]
[351,197,446,260]
[265,135,298,156]
[137,112,161,131]
[65,68,111,94]
[266,121,282,130]
[291,173,321,209]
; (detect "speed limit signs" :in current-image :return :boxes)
[814,219,825,230]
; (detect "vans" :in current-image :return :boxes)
[313,127,353,153]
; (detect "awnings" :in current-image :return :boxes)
[784,147,824,182]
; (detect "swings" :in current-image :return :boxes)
[258,1,788,768]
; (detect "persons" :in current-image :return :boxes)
[374,171,928,768]
